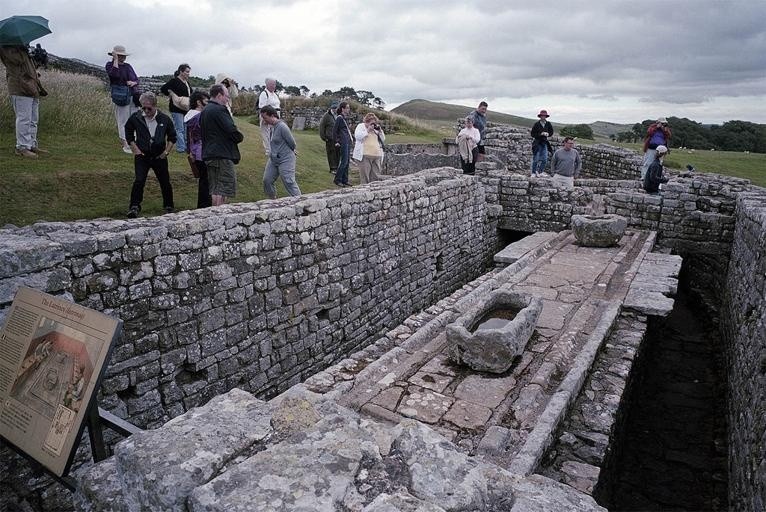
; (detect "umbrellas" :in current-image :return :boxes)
[0,14,52,47]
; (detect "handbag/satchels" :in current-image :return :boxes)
[111,85,130,106]
[173,96,190,111]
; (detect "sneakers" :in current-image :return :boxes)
[334,180,352,187]
[530,171,541,178]
[123,147,133,154]
[16,146,48,158]
[127,206,139,218]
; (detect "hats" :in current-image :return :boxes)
[656,117,668,124]
[538,111,549,118]
[656,145,668,154]
[108,45,129,57]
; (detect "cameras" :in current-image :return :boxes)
[370,122,380,130]
[661,121,668,126]
[31,44,50,69]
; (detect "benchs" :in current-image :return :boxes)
[12,342,82,414]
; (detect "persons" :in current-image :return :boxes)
[466,102,489,164]
[350,112,385,185]
[332,103,355,188]
[63,363,86,405]
[259,105,301,198]
[213,73,240,117]
[34,338,47,359]
[105,45,144,155]
[644,145,670,196]
[198,84,245,206]
[0,44,49,158]
[530,109,555,179]
[642,116,672,180]
[260,77,283,156]
[160,64,192,154]
[551,137,583,188]
[182,90,212,209]
[124,91,178,217]
[455,116,480,175]
[320,104,347,178]
